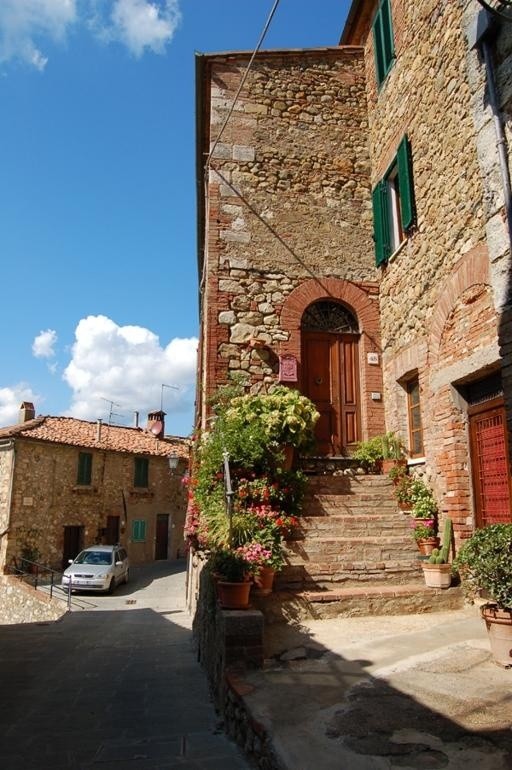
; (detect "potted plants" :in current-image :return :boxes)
[454,522,511,669]
[414,519,453,588]
[378,432,439,529]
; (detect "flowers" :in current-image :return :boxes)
[200,467,296,579]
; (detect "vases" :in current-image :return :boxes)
[242,565,274,588]
[216,582,252,610]
[411,517,436,533]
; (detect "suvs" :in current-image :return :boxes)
[62,545,128,594]
